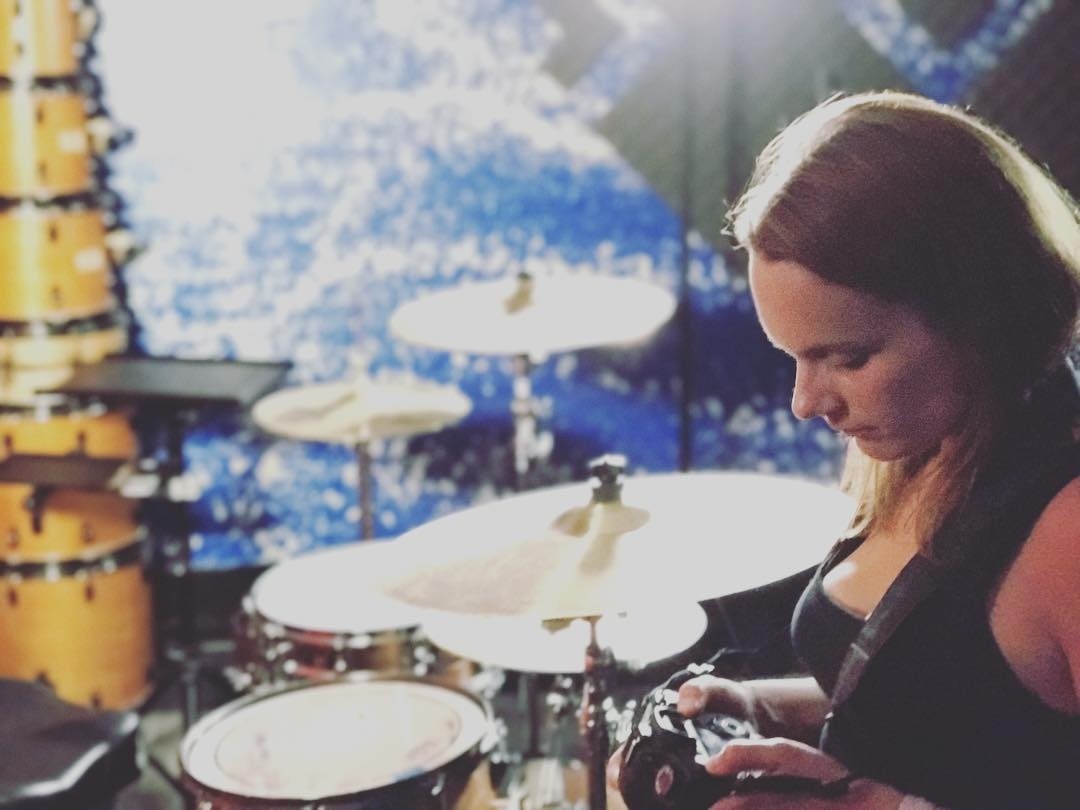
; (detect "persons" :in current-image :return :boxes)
[606,93,1080,810]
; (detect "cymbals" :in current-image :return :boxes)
[412,600,706,680]
[249,377,470,445]
[372,468,861,619]
[385,278,684,353]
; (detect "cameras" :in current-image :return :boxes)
[617,687,765,810]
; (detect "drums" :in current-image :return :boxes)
[171,539,510,810]
[0,0,163,716]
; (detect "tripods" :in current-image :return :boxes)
[33,354,295,809]
